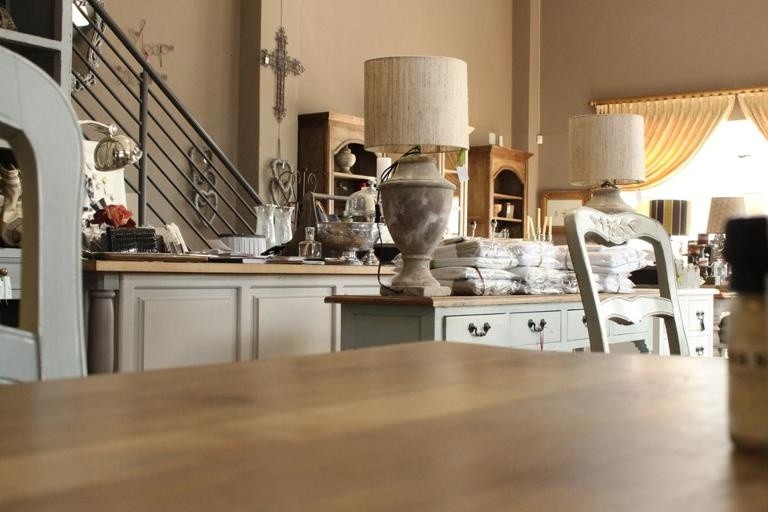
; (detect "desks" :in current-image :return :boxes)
[324,287,720,358]
[714,290,736,358]
[0,339,767,512]
[82,258,399,374]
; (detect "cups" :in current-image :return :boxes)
[713,259,728,286]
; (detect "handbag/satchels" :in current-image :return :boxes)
[0,164,24,248]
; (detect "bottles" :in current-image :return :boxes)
[274,206,295,247]
[723,214,768,453]
[297,226,322,259]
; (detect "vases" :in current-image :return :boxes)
[275,206,295,244]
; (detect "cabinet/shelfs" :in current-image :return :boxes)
[296,111,385,218]
[0,0,73,303]
[469,144,533,237]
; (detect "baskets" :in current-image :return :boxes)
[106,227,157,253]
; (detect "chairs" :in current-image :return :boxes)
[107,225,157,250]
[565,205,693,355]
[0,49,89,387]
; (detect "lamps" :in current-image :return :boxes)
[568,114,647,291]
[707,196,746,260]
[648,200,694,249]
[361,55,468,297]
[79,117,144,175]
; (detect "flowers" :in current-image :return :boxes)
[95,205,133,227]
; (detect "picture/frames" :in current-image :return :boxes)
[539,188,591,235]
